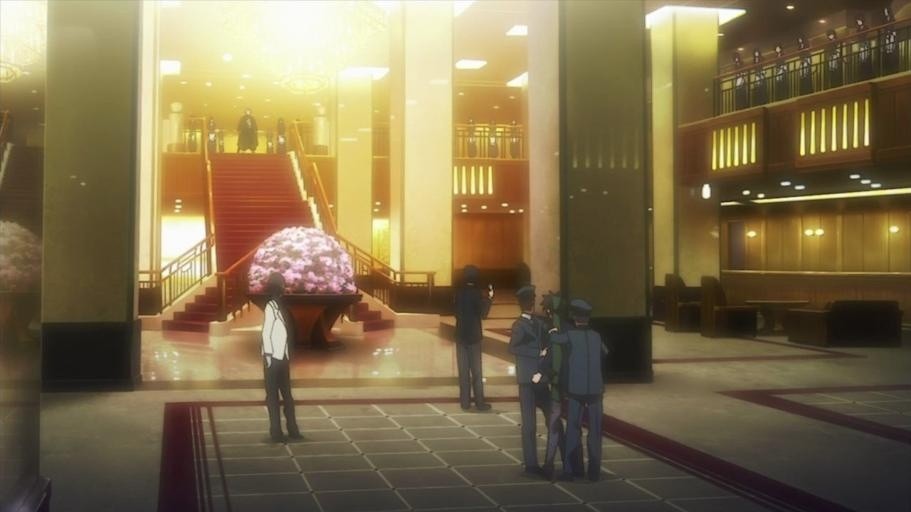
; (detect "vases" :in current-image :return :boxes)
[261,294,363,352]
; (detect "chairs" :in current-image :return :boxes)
[664,268,698,334]
[699,273,754,335]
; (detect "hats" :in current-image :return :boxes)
[571,299,593,315]
[514,285,537,299]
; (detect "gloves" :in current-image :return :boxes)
[265,355,272,370]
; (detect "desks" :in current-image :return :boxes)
[750,292,806,336]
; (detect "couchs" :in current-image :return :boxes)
[788,295,902,348]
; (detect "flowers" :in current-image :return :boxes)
[251,223,355,291]
[0,219,45,296]
